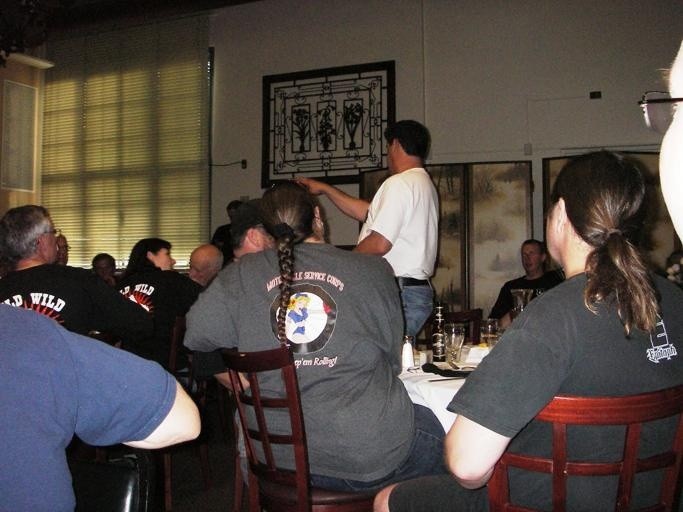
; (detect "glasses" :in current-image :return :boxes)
[44,227,60,238]
[637,89,683,136]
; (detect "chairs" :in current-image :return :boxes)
[174,330,238,493]
[226,347,382,512]
[484,386,681,512]
[415,307,482,357]
[64,438,165,511]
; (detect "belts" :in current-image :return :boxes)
[393,277,429,287]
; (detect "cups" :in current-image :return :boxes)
[403,335,415,368]
[443,322,466,363]
[480,319,498,345]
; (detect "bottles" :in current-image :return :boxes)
[432,302,446,362]
